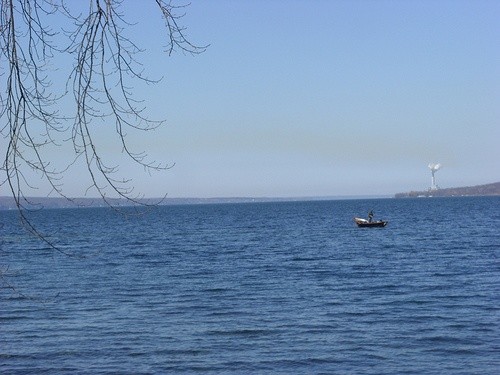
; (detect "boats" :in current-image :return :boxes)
[354,217,389,227]
